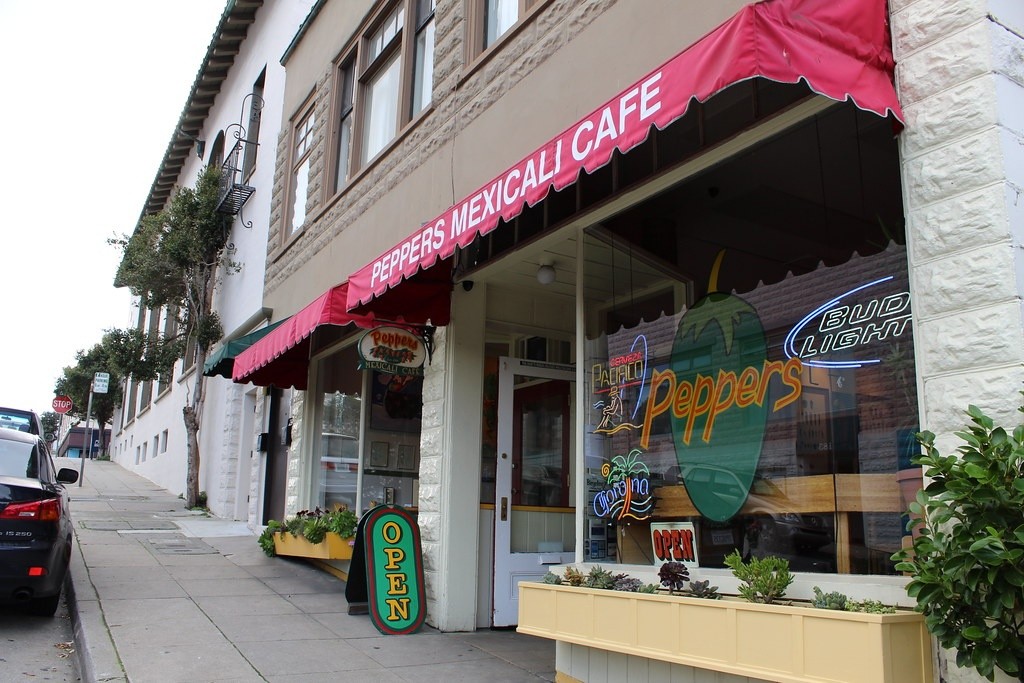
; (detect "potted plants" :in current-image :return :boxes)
[515,549,938,683]
[257,506,359,559]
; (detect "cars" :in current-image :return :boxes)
[0,427,80,619]
[0,407,57,443]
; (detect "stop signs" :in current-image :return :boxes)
[51,395,73,414]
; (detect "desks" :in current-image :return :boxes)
[614,472,916,575]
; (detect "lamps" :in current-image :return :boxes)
[537,265,557,284]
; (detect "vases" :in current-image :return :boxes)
[898,468,925,541]
